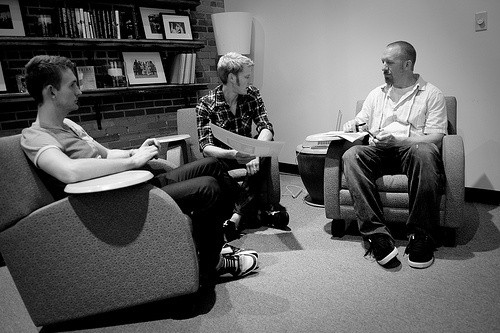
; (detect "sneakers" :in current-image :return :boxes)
[403,228,435,268]
[220,243,258,258]
[363,233,398,265]
[216,254,257,276]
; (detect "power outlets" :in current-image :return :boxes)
[472,11,489,31]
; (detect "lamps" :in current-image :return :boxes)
[208,12,254,56]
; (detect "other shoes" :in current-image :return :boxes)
[224,219,240,242]
[261,210,289,229]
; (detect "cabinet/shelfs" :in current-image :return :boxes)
[0,0,213,88]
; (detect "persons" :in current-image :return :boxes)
[133,58,156,75]
[20,54,259,277]
[170,22,185,34]
[342,41,448,268]
[197,52,289,231]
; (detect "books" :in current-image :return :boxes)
[59,6,141,40]
[169,52,197,84]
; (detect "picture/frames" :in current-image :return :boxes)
[122,50,170,85]
[0,0,26,36]
[136,6,194,40]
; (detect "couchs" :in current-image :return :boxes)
[320,96,467,234]
[0,108,281,328]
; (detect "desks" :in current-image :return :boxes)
[295,117,346,209]
